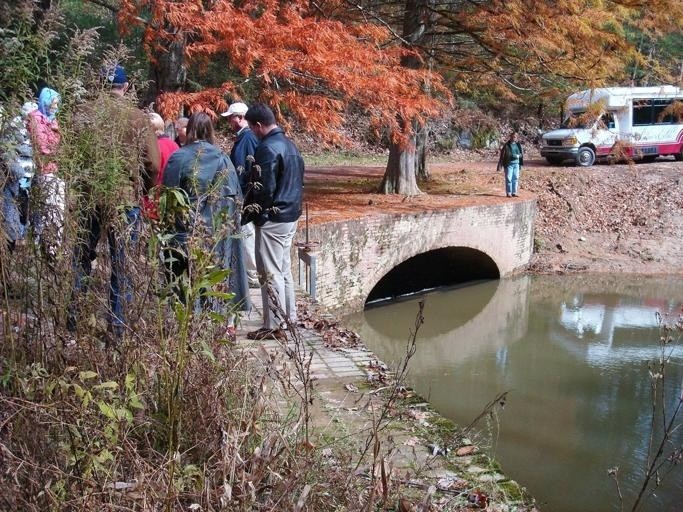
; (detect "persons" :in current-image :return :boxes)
[495,133,523,197]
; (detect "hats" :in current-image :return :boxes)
[19,101,39,118]
[220,101,249,117]
[98,65,128,86]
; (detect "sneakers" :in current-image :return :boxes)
[506,193,519,198]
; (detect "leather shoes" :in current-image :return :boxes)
[246,328,288,343]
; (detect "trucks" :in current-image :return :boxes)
[553,295,683,368]
[538,86,683,167]
